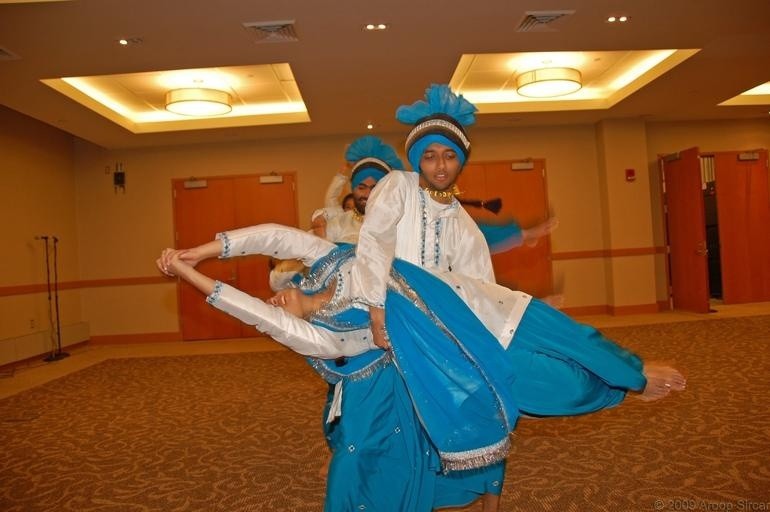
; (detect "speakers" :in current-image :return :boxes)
[114,172,125,185]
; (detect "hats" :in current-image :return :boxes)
[404,112,472,174]
[350,157,392,189]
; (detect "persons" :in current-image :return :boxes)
[156,114,686,512]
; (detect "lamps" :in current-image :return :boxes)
[515,67,583,99]
[164,86,233,117]
